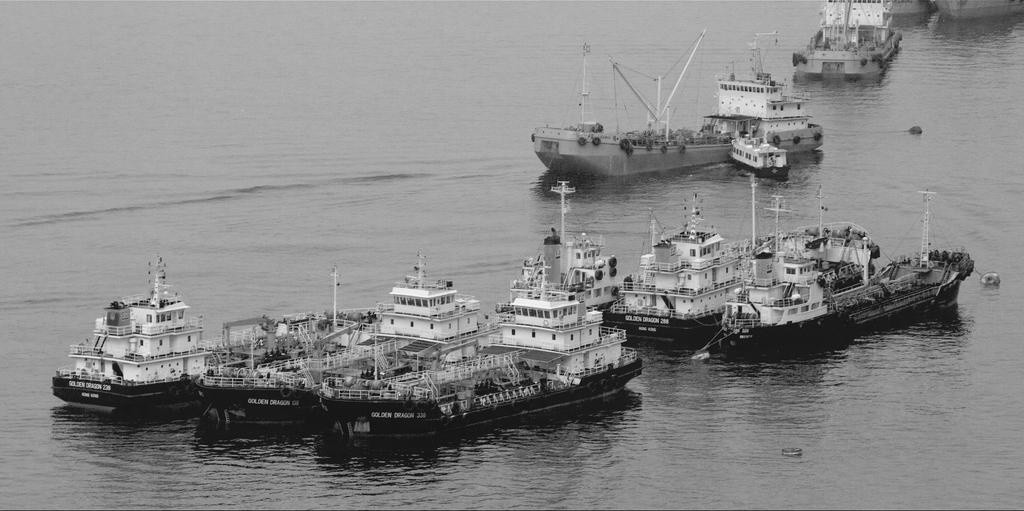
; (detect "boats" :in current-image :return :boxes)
[789,0,902,82]
[527,30,824,176]
[728,123,788,175]
